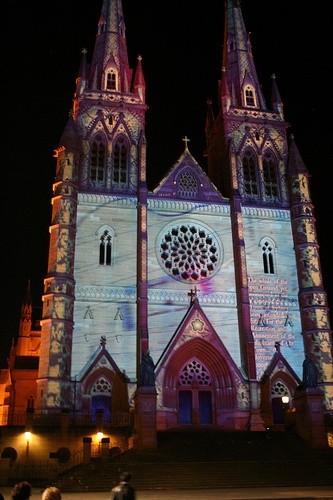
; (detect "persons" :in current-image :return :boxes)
[12,481,32,500]
[111,471,136,500]
[42,487,62,500]
